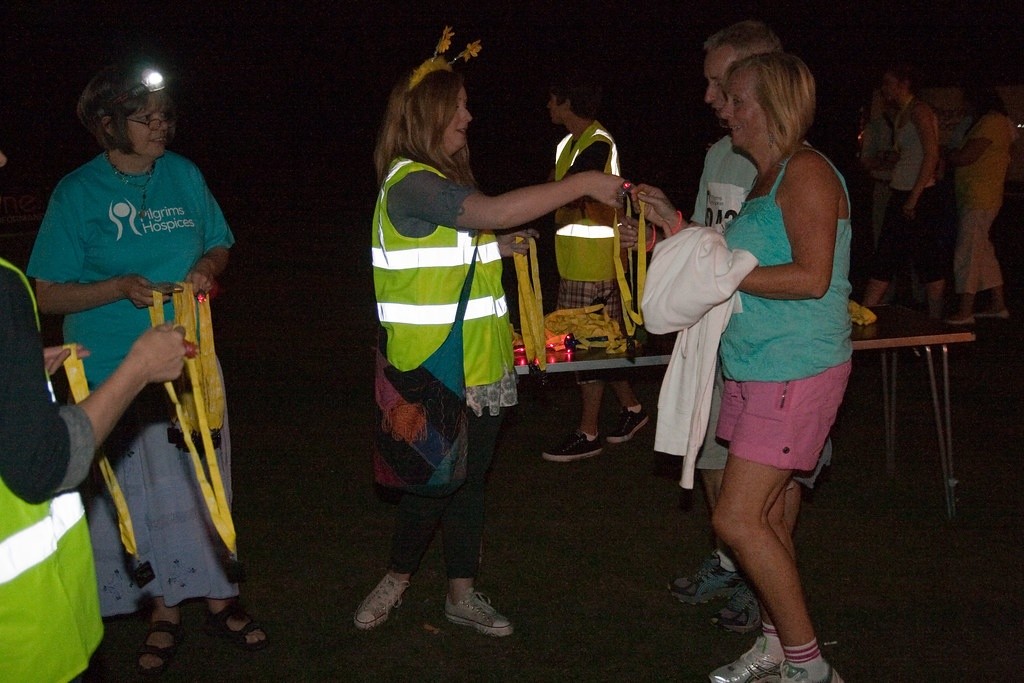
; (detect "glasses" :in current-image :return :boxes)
[121,116,177,132]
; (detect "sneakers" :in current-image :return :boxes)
[446,588,512,637]
[542,433,605,461]
[709,635,785,683]
[709,579,761,632]
[606,407,648,442]
[668,547,743,605]
[776,661,843,683]
[354,574,411,629]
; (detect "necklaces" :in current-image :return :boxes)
[104,151,157,217]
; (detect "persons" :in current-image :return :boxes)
[0,151,198,683]
[859,67,1021,325]
[541,70,650,462]
[353,70,638,636]
[618,20,832,634]
[631,53,852,683]
[27,62,271,678]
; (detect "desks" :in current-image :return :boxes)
[484,307,977,523]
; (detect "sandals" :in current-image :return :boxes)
[205,608,268,650]
[137,620,183,674]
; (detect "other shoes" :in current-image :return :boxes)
[976,307,1009,319]
[942,313,977,325]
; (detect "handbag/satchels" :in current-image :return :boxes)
[372,320,467,496]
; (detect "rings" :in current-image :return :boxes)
[196,289,207,303]
[621,191,625,195]
[622,180,632,189]
[184,340,198,359]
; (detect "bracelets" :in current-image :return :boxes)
[672,210,683,233]
[647,224,656,252]
